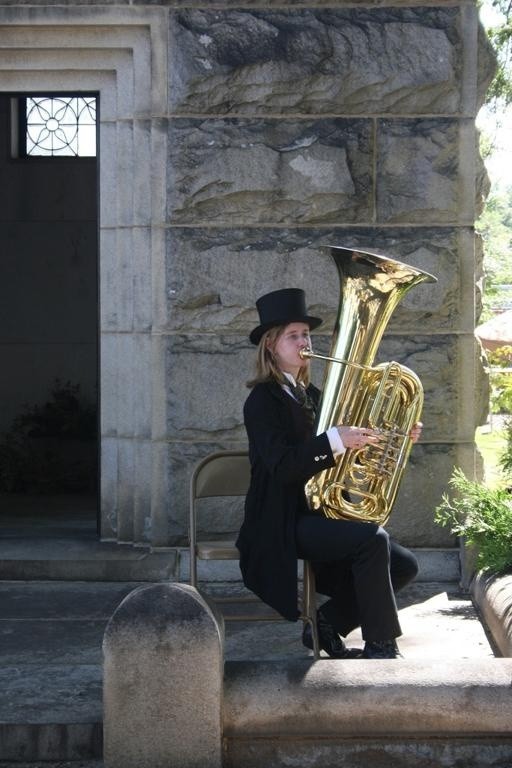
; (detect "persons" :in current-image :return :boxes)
[235,287,423,659]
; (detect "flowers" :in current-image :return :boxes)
[0,378,99,450]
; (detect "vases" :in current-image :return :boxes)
[30,432,98,475]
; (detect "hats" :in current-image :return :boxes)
[249,287,322,346]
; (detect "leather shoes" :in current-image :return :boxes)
[367,640,397,659]
[302,613,364,659]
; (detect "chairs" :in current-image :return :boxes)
[189,450,323,660]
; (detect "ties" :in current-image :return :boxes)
[280,372,318,419]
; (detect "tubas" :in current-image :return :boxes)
[296,242,439,525]
